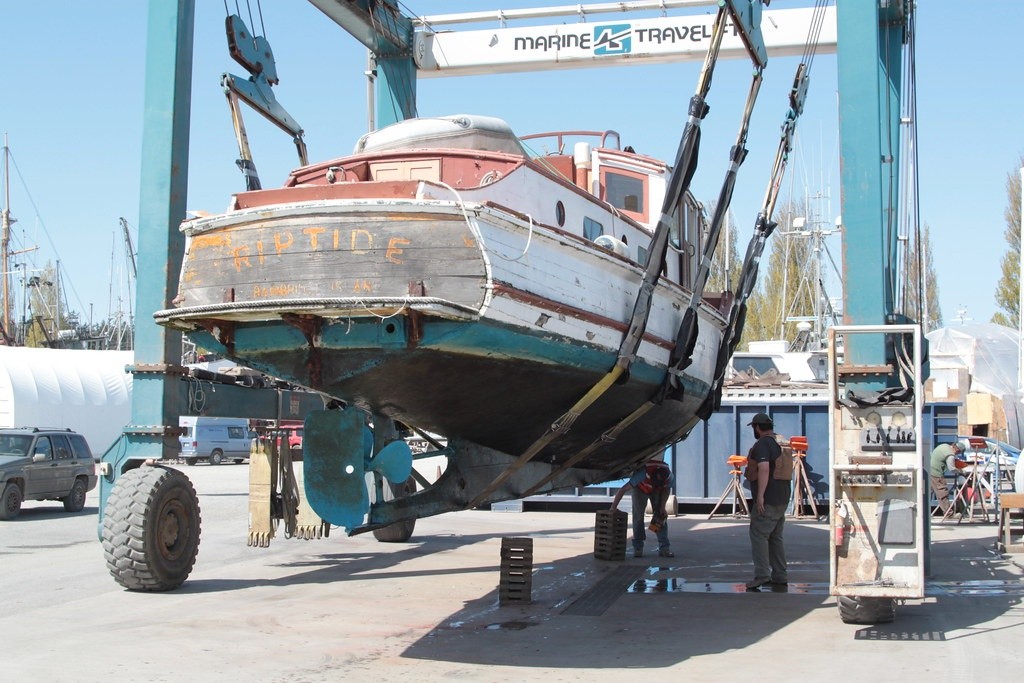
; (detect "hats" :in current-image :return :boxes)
[747,413,772,426]
[955,442,965,452]
[652,466,671,486]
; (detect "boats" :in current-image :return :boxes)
[151,114,735,537]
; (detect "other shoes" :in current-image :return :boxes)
[659,547,674,557]
[944,512,961,519]
[746,574,770,588]
[761,578,788,587]
[634,546,643,557]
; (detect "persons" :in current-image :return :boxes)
[610,460,673,557]
[930,442,965,519]
[743,413,793,588]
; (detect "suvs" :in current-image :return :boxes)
[0,427,98,520]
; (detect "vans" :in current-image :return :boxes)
[178,416,260,465]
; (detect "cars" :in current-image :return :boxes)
[959,436,1022,484]
[259,425,304,453]
